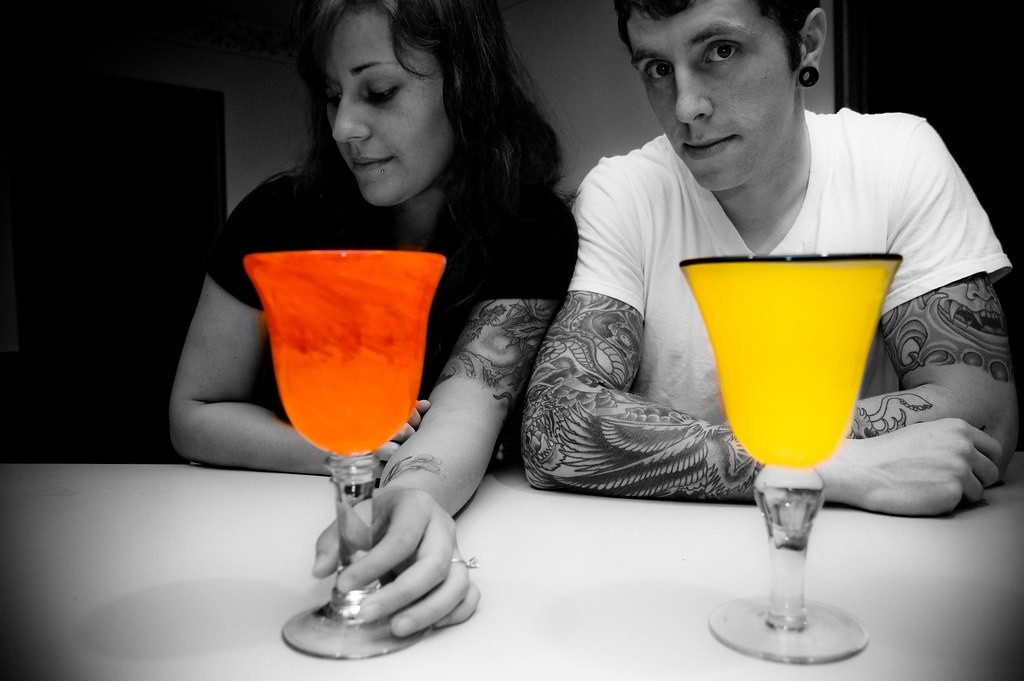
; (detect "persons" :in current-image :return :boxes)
[165,1,580,637]
[516,1,1018,514]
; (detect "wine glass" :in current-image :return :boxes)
[242,248,448,662]
[678,252,903,665]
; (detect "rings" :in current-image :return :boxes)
[451,557,479,569]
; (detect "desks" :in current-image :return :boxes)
[0,462,1024,681]
